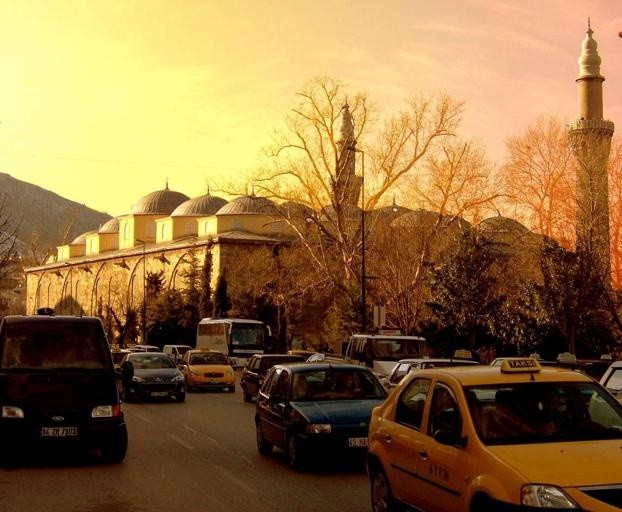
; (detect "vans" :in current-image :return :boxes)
[0,307,135,470]
[345,334,426,379]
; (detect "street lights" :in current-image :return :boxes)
[347,145,366,333]
[135,239,146,346]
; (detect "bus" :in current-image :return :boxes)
[195,317,273,370]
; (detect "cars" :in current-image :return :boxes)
[239,350,388,470]
[111,344,236,403]
